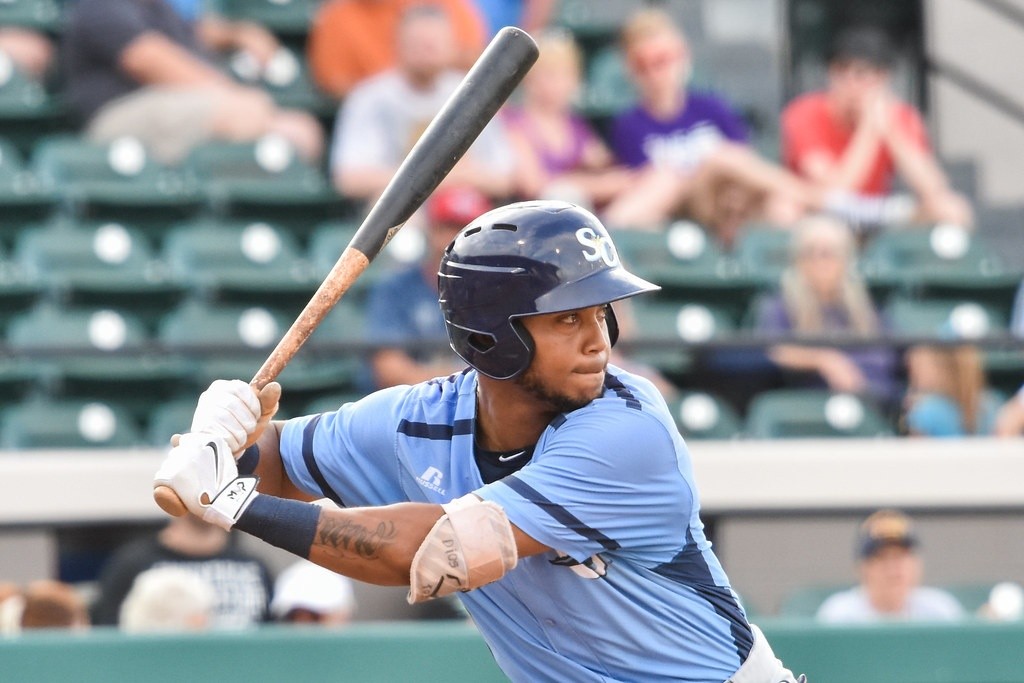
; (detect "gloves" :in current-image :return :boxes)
[190,379,281,463]
[153,429,263,533]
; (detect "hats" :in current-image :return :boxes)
[857,509,916,562]
[270,561,352,619]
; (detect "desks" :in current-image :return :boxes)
[0,620,1024,683]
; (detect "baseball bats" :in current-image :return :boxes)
[151,23,542,520]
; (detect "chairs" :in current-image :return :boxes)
[0,0,1024,434]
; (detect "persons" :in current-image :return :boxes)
[152,198,806,683]
[809,508,973,624]
[91,509,275,632]
[267,557,358,625]
[2,1,1024,439]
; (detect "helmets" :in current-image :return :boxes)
[438,200,665,381]
